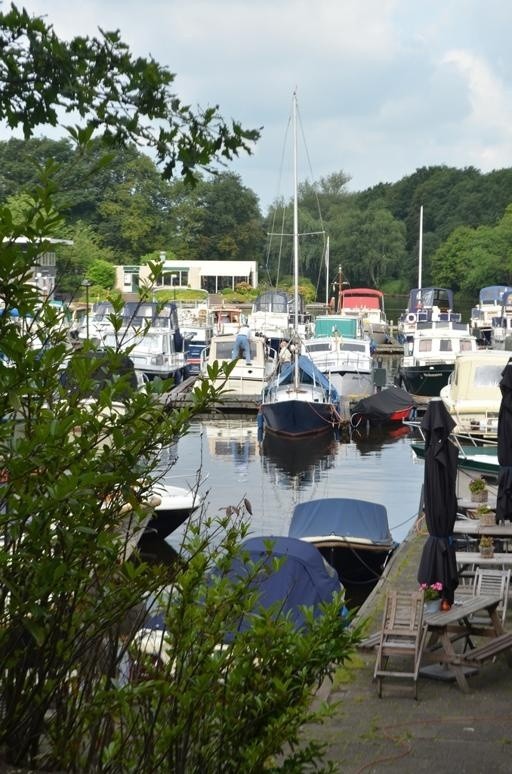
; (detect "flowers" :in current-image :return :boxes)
[416,582,443,599]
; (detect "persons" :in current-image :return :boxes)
[231,324,252,363]
[331,331,340,353]
[279,342,291,374]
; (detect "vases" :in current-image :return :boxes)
[425,598,443,612]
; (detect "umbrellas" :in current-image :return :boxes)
[421,397,460,607]
[495,357,512,525]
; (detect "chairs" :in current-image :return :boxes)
[372,581,428,703]
[463,566,510,664]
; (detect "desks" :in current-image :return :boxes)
[421,590,509,692]
[445,496,511,586]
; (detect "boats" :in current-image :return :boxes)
[127,536,349,695]
[257,437,344,505]
[347,428,410,460]
[203,416,260,485]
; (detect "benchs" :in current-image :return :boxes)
[445,633,511,693]
[355,630,383,650]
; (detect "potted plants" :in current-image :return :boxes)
[468,477,489,501]
